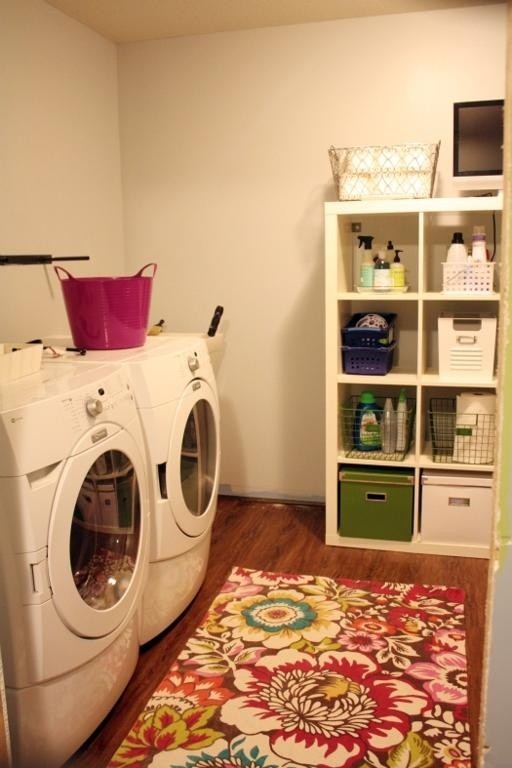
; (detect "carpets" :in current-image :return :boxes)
[107,563,470,763]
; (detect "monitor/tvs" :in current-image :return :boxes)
[450,99,504,195]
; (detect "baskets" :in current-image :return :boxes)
[54,262,159,350]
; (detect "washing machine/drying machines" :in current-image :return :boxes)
[24,333,220,648]
[0,363,151,766]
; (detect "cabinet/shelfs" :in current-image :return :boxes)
[325,197,503,559]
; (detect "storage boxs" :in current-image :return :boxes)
[421,470,492,546]
[438,311,496,379]
[328,141,441,200]
[339,470,414,543]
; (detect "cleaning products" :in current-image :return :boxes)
[396,390,408,452]
[355,393,383,451]
[378,398,397,454]
